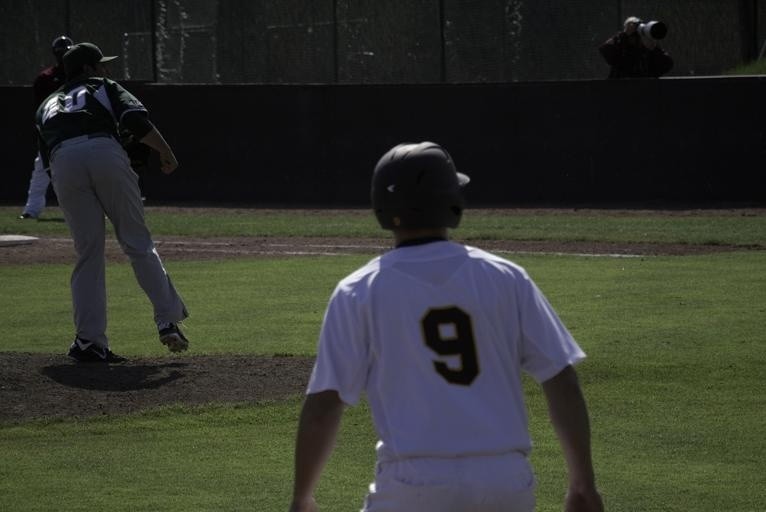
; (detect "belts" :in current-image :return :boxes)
[51,132,112,154]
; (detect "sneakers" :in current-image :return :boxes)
[68,336,130,364]
[158,321,189,352]
[18,213,33,220]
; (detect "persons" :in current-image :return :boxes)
[598,16,675,77]
[289,138,607,512]
[33,40,193,363]
[18,34,75,219]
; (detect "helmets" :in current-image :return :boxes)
[372,141,469,227]
[53,36,74,54]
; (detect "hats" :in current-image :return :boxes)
[62,43,119,70]
